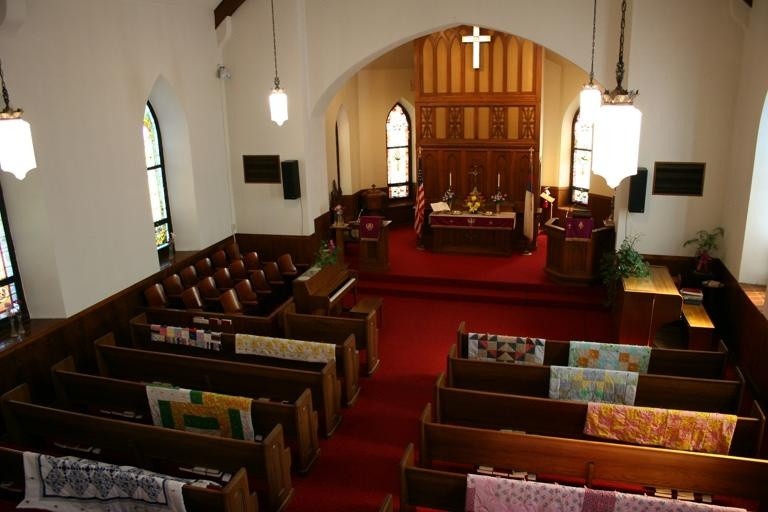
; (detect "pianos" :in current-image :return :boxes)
[293,262,359,316]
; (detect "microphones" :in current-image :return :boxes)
[355,209,364,223]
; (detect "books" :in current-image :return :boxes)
[679,286,706,305]
[430,201,452,213]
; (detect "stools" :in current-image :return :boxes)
[349,296,385,328]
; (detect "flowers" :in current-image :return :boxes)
[490,191,507,203]
[4,299,24,319]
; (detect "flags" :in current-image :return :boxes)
[413,159,426,238]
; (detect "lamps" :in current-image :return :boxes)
[577,0,644,190]
[0,68,37,181]
[268,1,289,127]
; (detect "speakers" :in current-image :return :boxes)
[281,160,301,199]
[628,168,647,213]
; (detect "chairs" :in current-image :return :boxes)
[143,243,299,313]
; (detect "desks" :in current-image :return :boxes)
[429,212,517,257]
[328,220,393,273]
[610,264,682,347]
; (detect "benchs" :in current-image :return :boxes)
[680,303,715,350]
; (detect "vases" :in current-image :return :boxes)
[17,314,26,334]
[9,319,18,338]
[495,203,501,214]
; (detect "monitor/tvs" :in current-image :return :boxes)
[652,162,706,196]
[243,155,280,183]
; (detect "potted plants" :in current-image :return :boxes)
[154,225,175,262]
[683,225,726,275]
